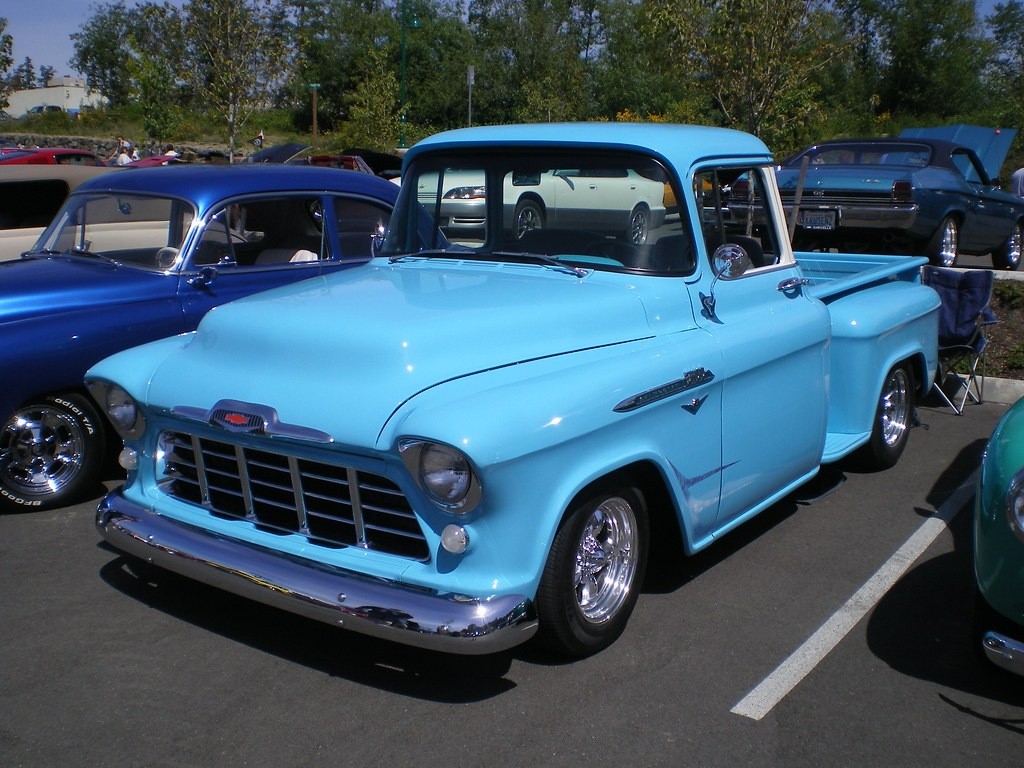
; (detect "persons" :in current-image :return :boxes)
[1009,167,1024,197]
[148,141,156,156]
[162,143,181,166]
[107,136,143,164]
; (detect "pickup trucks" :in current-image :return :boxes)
[82,121,945,659]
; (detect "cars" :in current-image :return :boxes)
[661,168,711,211]
[0,166,470,512]
[388,166,669,249]
[974,394,1024,679]
[0,140,406,237]
[0,164,266,266]
[725,127,1024,271]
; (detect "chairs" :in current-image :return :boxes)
[244,244,318,265]
[515,228,615,260]
[651,235,765,271]
[916,264,1002,416]
[193,241,228,265]
